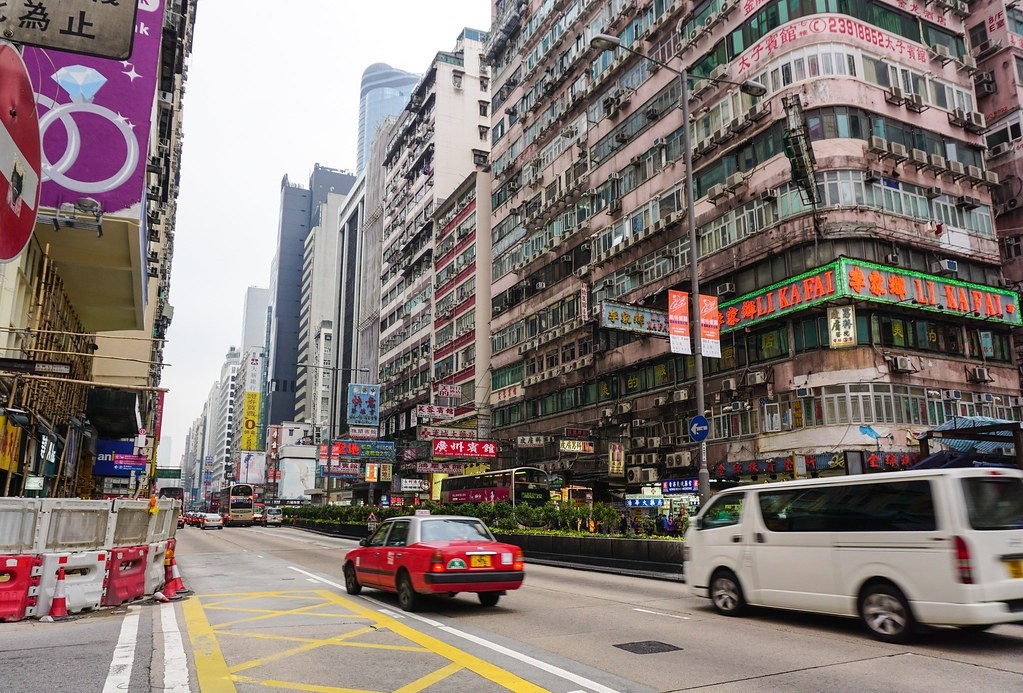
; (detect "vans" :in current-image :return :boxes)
[266,508,283,527]
[682,466,1023,643]
[253,503,265,525]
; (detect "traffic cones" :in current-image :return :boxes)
[49,568,74,620]
[170,559,190,593]
[162,565,182,599]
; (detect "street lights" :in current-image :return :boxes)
[296,362,370,506]
[589,33,768,511]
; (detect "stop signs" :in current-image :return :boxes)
[0,42,41,263]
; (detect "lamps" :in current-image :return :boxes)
[9,409,92,447]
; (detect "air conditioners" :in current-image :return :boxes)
[887,254,898,264]
[959,55,977,71]
[147,2,195,408]
[975,72,993,96]
[866,136,998,209]
[1005,195,1023,211]
[1012,397,1023,405]
[887,86,925,110]
[943,389,962,401]
[999,278,1010,286]
[936,0,969,16]
[893,356,911,371]
[992,142,1009,156]
[971,368,988,380]
[975,393,992,403]
[953,107,987,131]
[980,38,997,51]
[931,259,958,275]
[931,44,950,61]
[380,0,814,484]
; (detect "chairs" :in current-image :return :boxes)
[759,498,779,531]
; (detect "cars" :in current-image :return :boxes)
[341,510,525,613]
[177,512,205,528]
[177,514,184,529]
[202,514,223,530]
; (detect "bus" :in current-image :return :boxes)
[219,484,253,527]
[440,466,550,510]
[159,487,183,515]
[208,491,221,514]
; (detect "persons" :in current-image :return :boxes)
[656,514,683,537]
[620,514,628,534]
[646,518,654,536]
[632,517,640,535]
[487,490,495,505]
[241,455,263,482]
